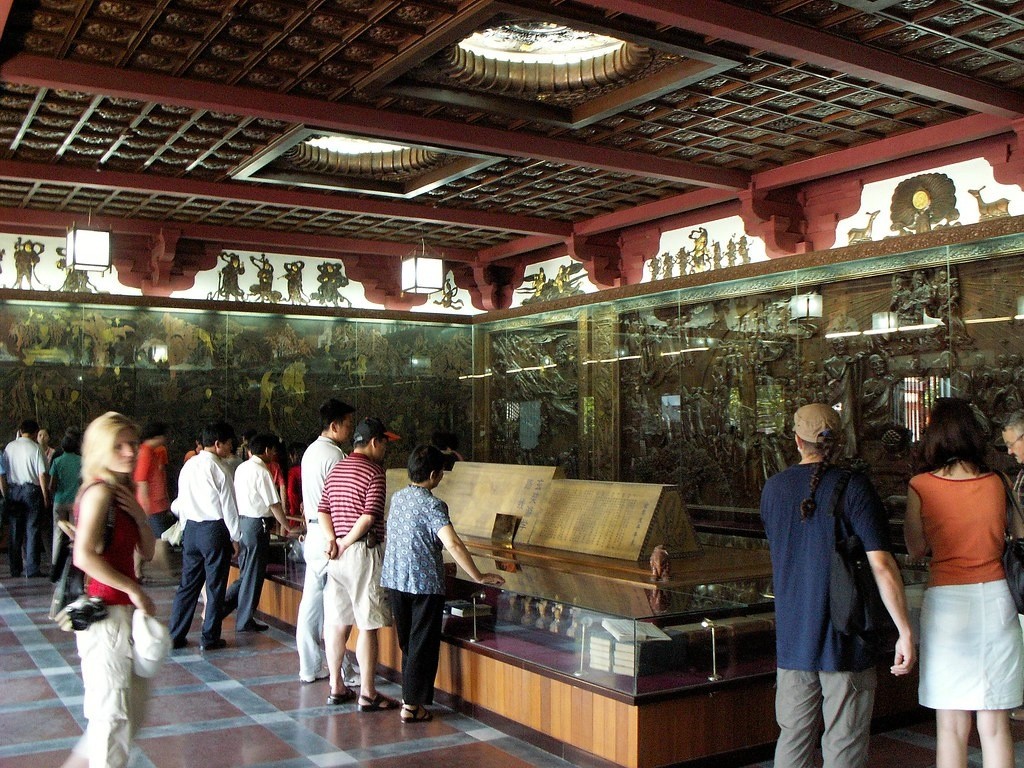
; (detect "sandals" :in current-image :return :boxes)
[400,702,433,722]
[326,686,358,706]
[357,691,402,711]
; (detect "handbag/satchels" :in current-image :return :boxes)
[984,464,1024,616]
[46,555,85,619]
[829,464,891,633]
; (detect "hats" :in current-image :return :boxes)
[130,608,170,678]
[353,417,401,448]
[792,404,842,442]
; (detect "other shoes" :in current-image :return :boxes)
[26,570,47,578]
[254,623,270,631]
[200,639,227,650]
[135,573,148,585]
[171,637,189,649]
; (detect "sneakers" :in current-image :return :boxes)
[340,665,363,686]
[299,666,330,683]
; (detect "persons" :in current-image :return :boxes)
[1003,409,1024,720]
[759,404,917,768]
[318,416,401,712]
[380,446,506,725]
[166,423,228,651]
[58,413,156,768]
[223,435,293,633]
[904,400,1023,768]
[295,401,358,686]
[0,417,306,620]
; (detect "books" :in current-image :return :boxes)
[589,612,776,676]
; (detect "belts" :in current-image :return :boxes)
[335,533,366,542]
[309,518,319,524]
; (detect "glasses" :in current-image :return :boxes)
[1005,431,1024,451]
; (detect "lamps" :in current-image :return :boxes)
[791,294,823,320]
[872,310,897,332]
[151,344,168,364]
[65,191,112,277]
[400,223,444,300]
